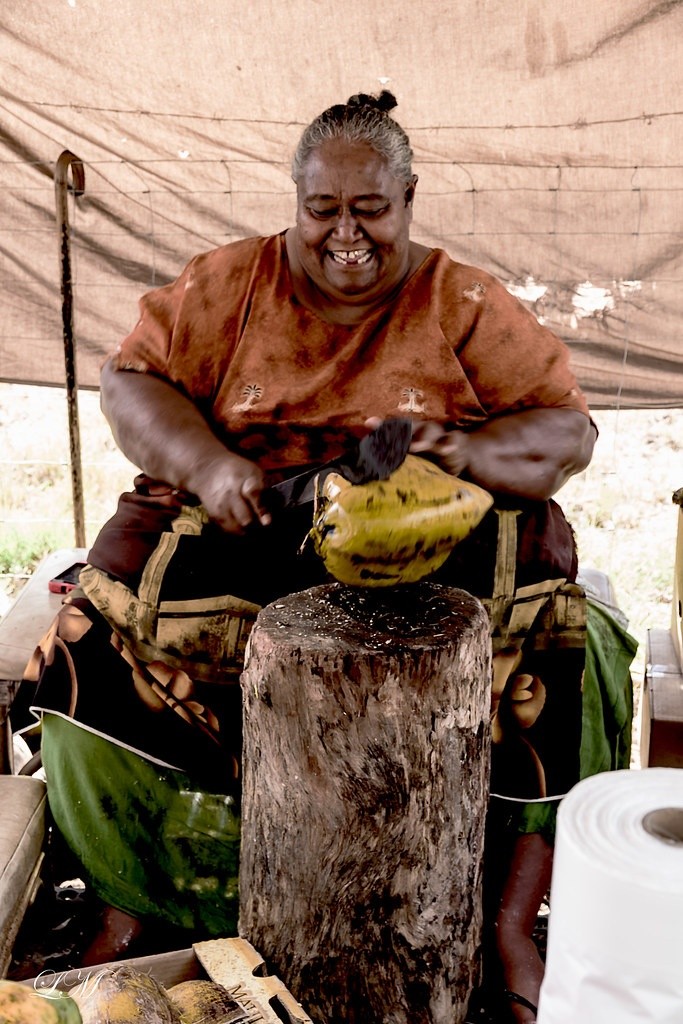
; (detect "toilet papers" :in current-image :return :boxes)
[532,766,683,1023]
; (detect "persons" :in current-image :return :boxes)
[7,87,599,1023]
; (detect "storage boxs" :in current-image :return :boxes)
[16,937,313,1024]
[639,629,683,769]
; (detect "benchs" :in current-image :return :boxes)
[0,550,614,775]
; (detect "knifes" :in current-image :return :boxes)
[208,416,414,509]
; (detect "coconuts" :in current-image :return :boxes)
[0,966,243,1024]
